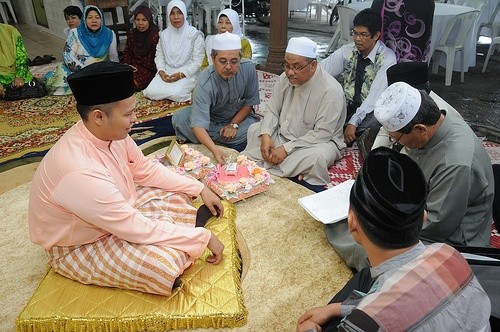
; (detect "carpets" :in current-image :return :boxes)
[0,60,193,163]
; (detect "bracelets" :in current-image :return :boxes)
[179,72,182,79]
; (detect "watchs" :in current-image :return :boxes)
[230,122,239,130]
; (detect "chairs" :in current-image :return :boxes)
[325,6,360,56]
[433,10,482,86]
[305,0,331,23]
[198,0,220,34]
[477,2,500,73]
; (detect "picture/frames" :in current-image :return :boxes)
[165,138,185,165]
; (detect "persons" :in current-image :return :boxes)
[0,23,46,104]
[200,9,252,72]
[38,5,121,96]
[27,61,225,298]
[118,5,160,93]
[239,36,347,190]
[172,32,261,167]
[141,0,207,102]
[295,145,492,332]
[370,61,495,248]
[370,0,436,94]
[317,8,397,148]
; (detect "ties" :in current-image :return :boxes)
[352,53,371,108]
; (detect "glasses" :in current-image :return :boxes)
[280,59,314,71]
[351,29,372,38]
[380,126,404,146]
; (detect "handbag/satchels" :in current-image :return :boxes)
[3,77,46,101]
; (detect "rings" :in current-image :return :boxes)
[19,82,23,85]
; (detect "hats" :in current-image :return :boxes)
[285,37,317,58]
[373,82,421,132]
[212,31,241,51]
[349,146,429,231]
[67,60,135,106]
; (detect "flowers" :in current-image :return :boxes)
[151,144,211,174]
[207,154,270,194]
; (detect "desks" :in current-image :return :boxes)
[347,1,482,71]
